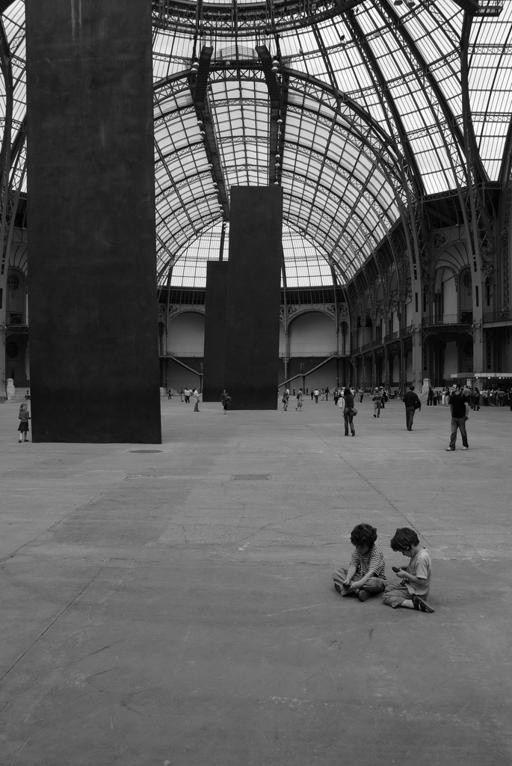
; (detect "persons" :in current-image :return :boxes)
[219,388,228,415]
[332,523,385,603]
[17,403,31,442]
[382,527,434,613]
[281,380,512,451]
[181,387,201,412]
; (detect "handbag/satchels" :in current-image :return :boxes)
[350,408,357,416]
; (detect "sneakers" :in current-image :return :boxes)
[446,446,468,450]
[334,582,367,601]
[345,430,355,437]
[414,596,434,613]
